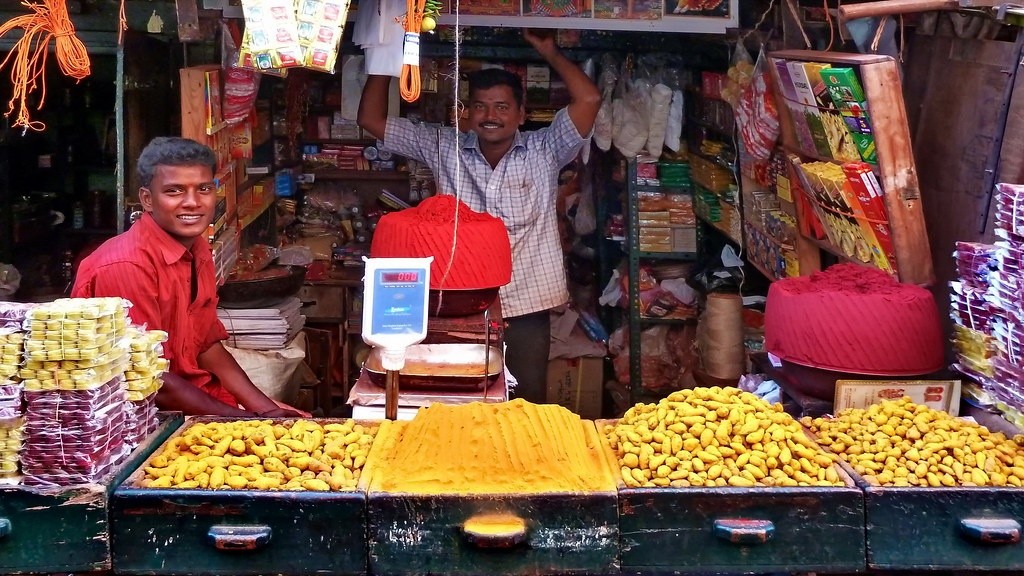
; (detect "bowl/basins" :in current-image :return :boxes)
[764,349,943,403]
[429,286,498,316]
[216,264,306,309]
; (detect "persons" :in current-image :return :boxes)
[71,136,305,417]
[357,26,604,407]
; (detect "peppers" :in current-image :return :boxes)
[424,0,444,18]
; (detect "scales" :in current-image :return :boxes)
[347,343,508,421]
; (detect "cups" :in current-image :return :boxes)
[90,190,105,228]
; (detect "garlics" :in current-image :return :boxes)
[421,16,436,34]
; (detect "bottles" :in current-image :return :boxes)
[84,84,92,107]
[73,202,84,229]
[350,206,362,216]
[369,218,379,230]
[356,230,370,243]
[64,88,71,106]
[352,216,367,230]
